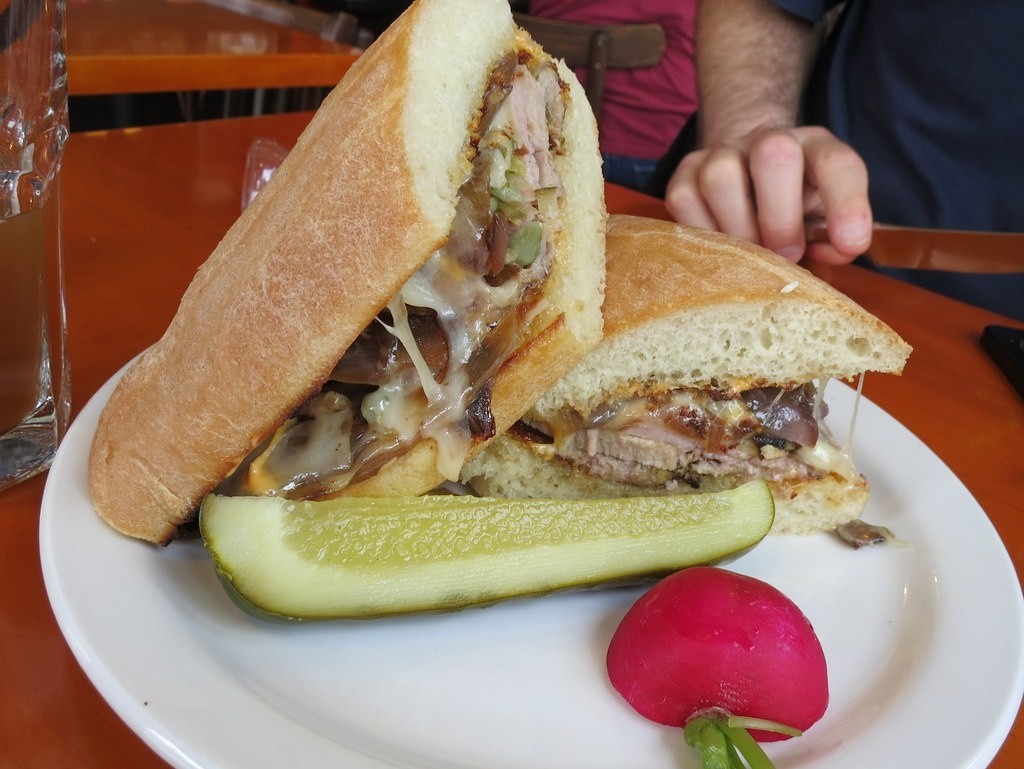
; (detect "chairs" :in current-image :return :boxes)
[511,14,668,124]
[185,1,361,123]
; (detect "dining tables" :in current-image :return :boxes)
[0,111,1024,769]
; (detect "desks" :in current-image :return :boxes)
[0,0,366,102]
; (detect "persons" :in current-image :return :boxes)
[511,0,1024,265]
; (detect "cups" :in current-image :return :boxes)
[0,0,72,494]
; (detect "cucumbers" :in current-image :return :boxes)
[198,477,775,623]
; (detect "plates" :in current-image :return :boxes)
[39,342,1024,769]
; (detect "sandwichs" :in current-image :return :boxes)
[87,0,610,548]
[441,212,913,533]
